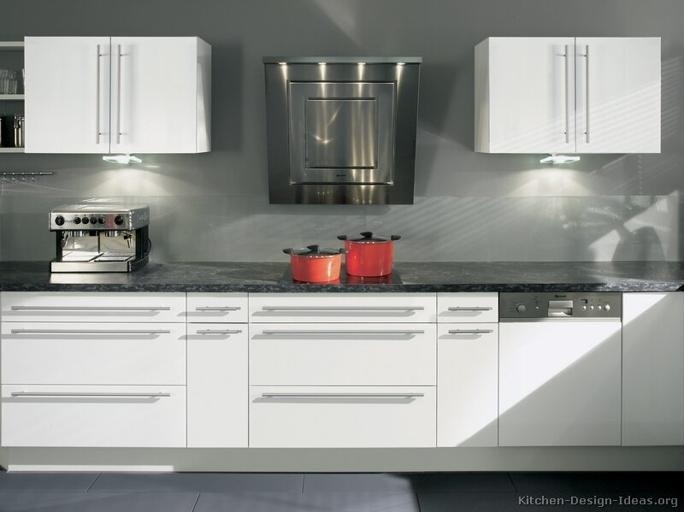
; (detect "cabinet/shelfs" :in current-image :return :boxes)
[473,36,662,156]
[0,37,212,155]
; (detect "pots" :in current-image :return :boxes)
[280,242,350,285]
[336,229,401,278]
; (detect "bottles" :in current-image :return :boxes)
[1,70,19,94]
[0,115,24,148]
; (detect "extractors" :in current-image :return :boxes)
[262,56,423,205]
